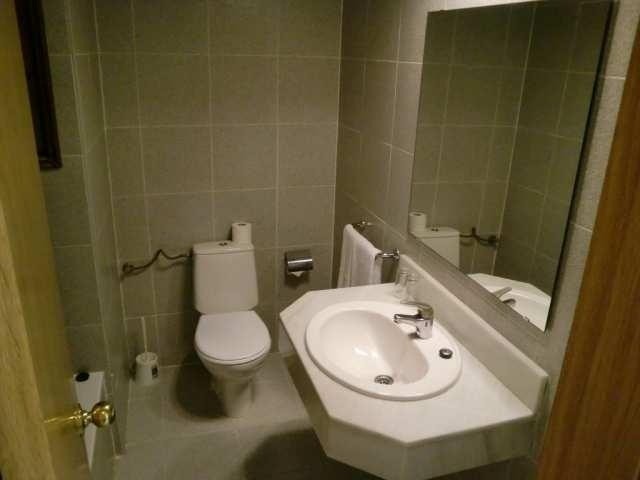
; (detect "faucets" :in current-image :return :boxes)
[493,286,517,305]
[394,301,435,340]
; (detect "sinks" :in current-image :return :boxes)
[465,274,552,332]
[303,302,461,400]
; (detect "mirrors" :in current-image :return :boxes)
[407,0,618,334]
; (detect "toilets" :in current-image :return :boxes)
[420,225,460,270]
[191,240,272,418]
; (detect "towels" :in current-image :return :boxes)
[337,223,384,287]
